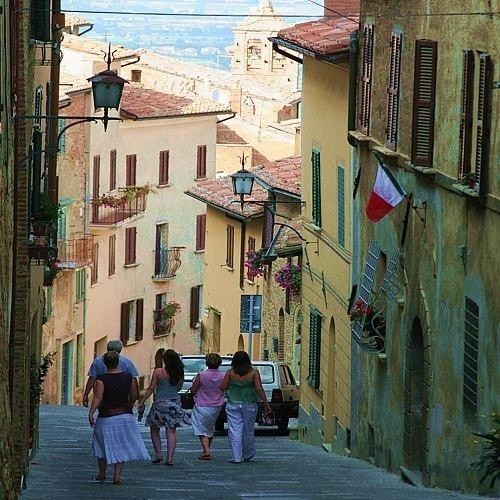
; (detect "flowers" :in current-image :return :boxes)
[243,248,267,279]
[273,263,302,295]
[49,258,66,277]
[349,299,385,336]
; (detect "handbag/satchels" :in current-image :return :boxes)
[180,387,195,409]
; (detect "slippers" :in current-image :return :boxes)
[198,455,211,460]
[165,460,174,465]
[151,459,163,464]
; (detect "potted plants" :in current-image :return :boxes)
[33,193,64,247]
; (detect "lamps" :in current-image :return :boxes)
[14,41,131,170]
[229,150,306,242]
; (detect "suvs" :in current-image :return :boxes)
[204,361,300,433]
[179,355,233,396]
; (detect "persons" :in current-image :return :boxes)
[139,349,191,465]
[83,341,138,405]
[89,351,151,484]
[221,352,272,463]
[189,353,226,460]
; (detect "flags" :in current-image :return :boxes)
[364,161,406,223]
